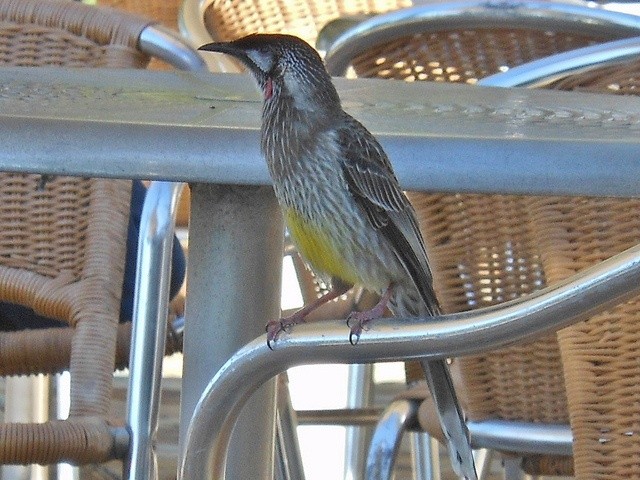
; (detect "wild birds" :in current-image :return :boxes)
[196,32,479,480]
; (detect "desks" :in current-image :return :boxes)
[5,67,640,480]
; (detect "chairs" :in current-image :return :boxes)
[2,1,214,480]
[172,40,639,480]
[322,0,640,479]
